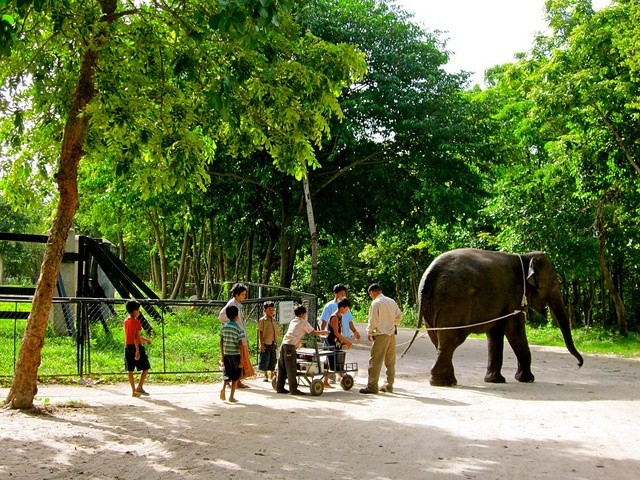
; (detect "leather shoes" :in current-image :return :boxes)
[381,387,393,392]
[360,388,378,394]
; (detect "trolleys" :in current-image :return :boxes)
[271,335,358,396]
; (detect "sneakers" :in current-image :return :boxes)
[135,387,149,396]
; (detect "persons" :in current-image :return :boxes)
[258,301,279,382]
[359,283,402,394]
[324,299,353,388]
[275,306,331,395]
[123,300,152,397]
[220,306,245,402]
[218,283,256,388]
[320,283,361,384]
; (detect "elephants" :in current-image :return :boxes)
[400,248,584,386]
